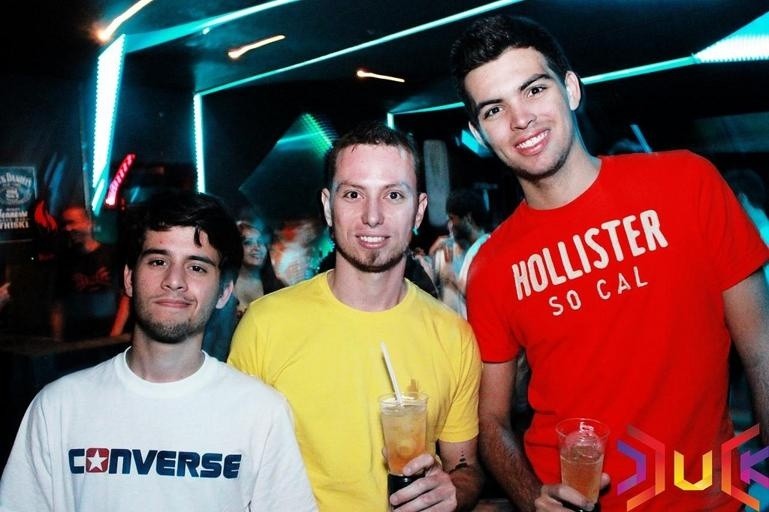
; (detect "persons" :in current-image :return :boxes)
[1,139,769,511]
[228,119,485,512]
[441,10,768,512]
